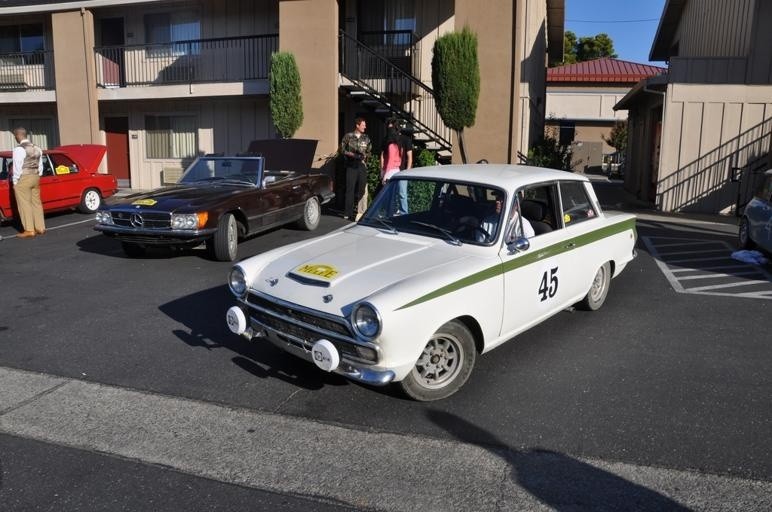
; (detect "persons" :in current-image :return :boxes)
[372,126,404,216]
[340,117,372,222]
[476,189,535,244]
[12,126,46,238]
[380,119,413,217]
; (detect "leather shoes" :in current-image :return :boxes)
[16,231,35,238]
[36,230,47,236]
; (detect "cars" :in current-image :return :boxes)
[225,165,640,403]
[93,139,336,262]
[736,183,772,259]
[0,141,122,216]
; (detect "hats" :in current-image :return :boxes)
[491,191,522,200]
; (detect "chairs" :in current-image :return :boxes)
[521,200,553,234]
